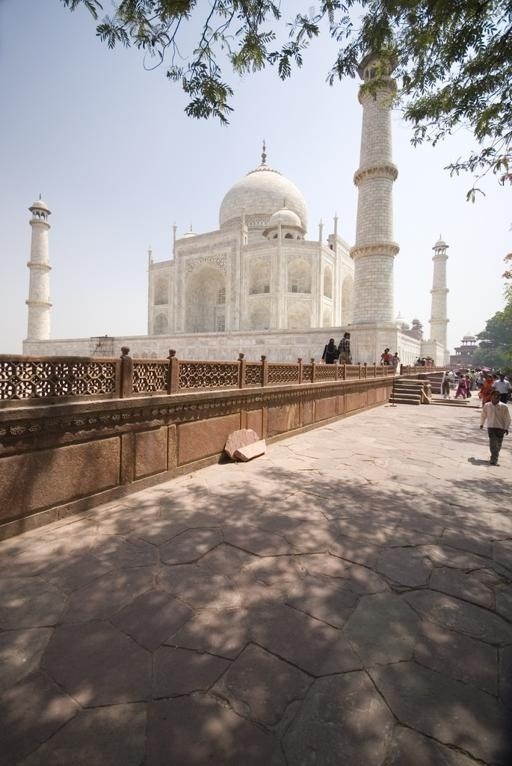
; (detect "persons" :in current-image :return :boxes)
[338,332,352,365]
[479,391,511,465]
[380,348,400,371]
[322,339,340,364]
[417,357,435,368]
[442,367,511,408]
